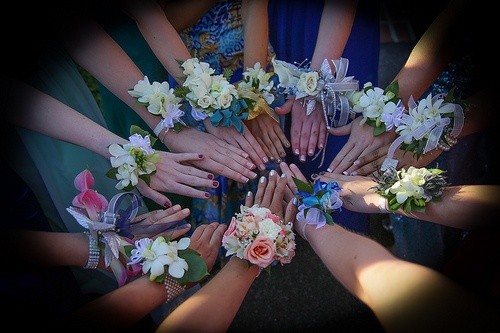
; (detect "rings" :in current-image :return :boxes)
[380,157,399,171]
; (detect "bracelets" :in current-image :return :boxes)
[271,54,359,168]
[222,204,296,278]
[105,125,160,192]
[291,176,344,241]
[128,236,210,304]
[161,127,170,144]
[128,75,191,136]
[66,168,189,287]
[388,92,464,158]
[367,164,448,214]
[236,62,280,124]
[353,81,409,136]
[175,57,250,133]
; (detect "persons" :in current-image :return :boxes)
[10,0,500,333]
